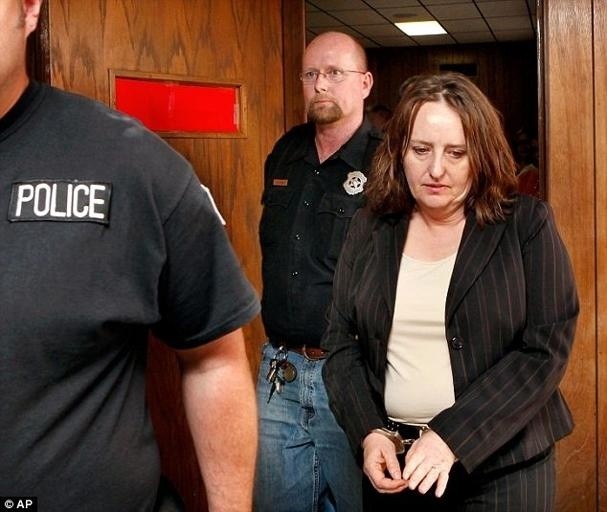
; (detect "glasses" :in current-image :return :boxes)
[299,68,365,83]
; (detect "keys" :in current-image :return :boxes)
[266,357,287,394]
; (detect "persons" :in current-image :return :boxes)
[507,122,539,200]
[319,67,580,511]
[362,102,393,142]
[254,28,391,510]
[0,0,269,511]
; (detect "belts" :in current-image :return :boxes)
[388,417,431,445]
[269,340,329,361]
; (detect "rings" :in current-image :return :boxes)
[429,465,440,474]
